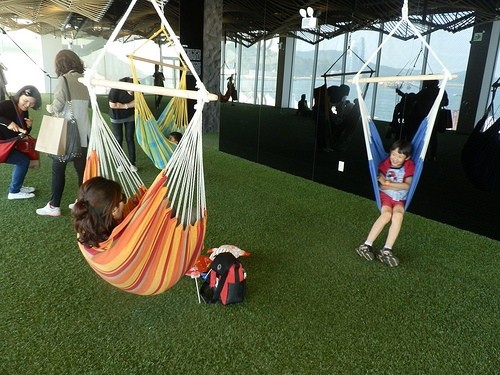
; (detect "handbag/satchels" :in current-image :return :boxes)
[436,109,453,128]
[46,74,82,163]
[34,109,67,155]
[15,134,39,161]
[231,83,237,100]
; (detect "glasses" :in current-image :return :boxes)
[102,192,127,218]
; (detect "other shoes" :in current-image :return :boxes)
[375,248,400,267]
[355,242,375,261]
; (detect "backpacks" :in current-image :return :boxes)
[199,252,248,305]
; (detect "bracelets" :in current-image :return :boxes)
[125,104,128,109]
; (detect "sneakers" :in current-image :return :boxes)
[116,164,140,173]
[35,201,62,217]
[7,184,36,200]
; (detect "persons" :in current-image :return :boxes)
[419,80,448,160]
[71,176,197,248]
[297,84,353,152]
[153,64,165,109]
[108,77,144,172]
[356,139,416,267]
[225,76,238,106]
[0,85,42,200]
[167,132,183,145]
[36,50,89,216]
